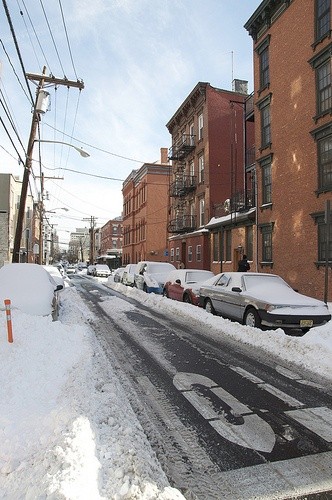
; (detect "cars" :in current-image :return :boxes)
[199,271,331,336]
[92,264,112,278]
[113,268,125,283]
[134,261,177,295]
[87,265,94,275]
[123,264,139,287]
[40,265,64,292]
[0,263,64,324]
[67,266,76,275]
[163,268,216,305]
[77,263,87,270]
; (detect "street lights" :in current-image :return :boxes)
[11,139,92,262]
[38,206,68,264]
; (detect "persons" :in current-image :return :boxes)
[237,255,250,272]
